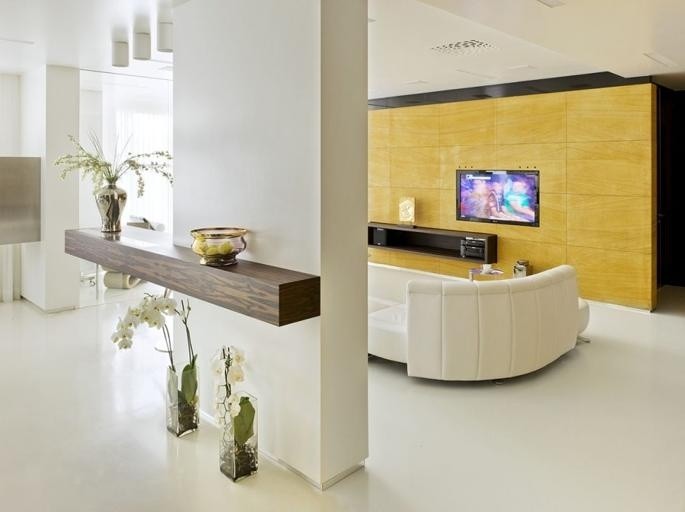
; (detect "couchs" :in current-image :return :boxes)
[367,262,591,386]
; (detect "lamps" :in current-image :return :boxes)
[110,21,174,67]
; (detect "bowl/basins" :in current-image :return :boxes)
[191,227,247,267]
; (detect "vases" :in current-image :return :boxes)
[93,184,127,233]
[215,392,260,485]
[164,365,200,437]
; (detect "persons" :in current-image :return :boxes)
[460,174,536,222]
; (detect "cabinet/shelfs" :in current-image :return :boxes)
[367,221,497,265]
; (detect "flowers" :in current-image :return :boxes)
[109,285,198,424]
[53,127,175,228]
[213,343,258,474]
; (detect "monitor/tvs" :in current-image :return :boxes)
[456,169,540,228]
